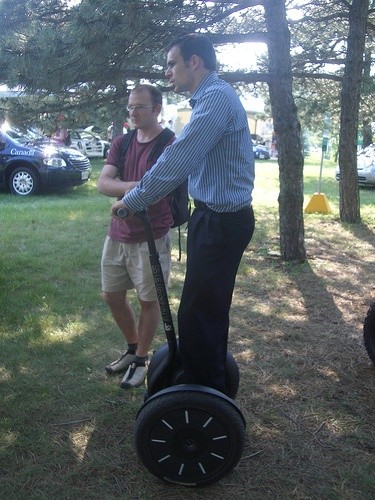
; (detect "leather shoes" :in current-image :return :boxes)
[105,350,136,375]
[120,363,148,389]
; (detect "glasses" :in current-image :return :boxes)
[125,105,156,111]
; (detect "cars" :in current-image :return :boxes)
[251,133,273,160]
[335,140,375,189]
[0,116,92,197]
[69,125,110,159]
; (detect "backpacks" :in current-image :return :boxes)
[64,134,71,146]
[118,128,190,228]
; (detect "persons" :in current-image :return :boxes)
[111,33,255,397]
[53,122,68,145]
[97,85,176,388]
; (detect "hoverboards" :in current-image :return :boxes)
[115,189,248,489]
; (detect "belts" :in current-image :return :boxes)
[194,200,205,210]
